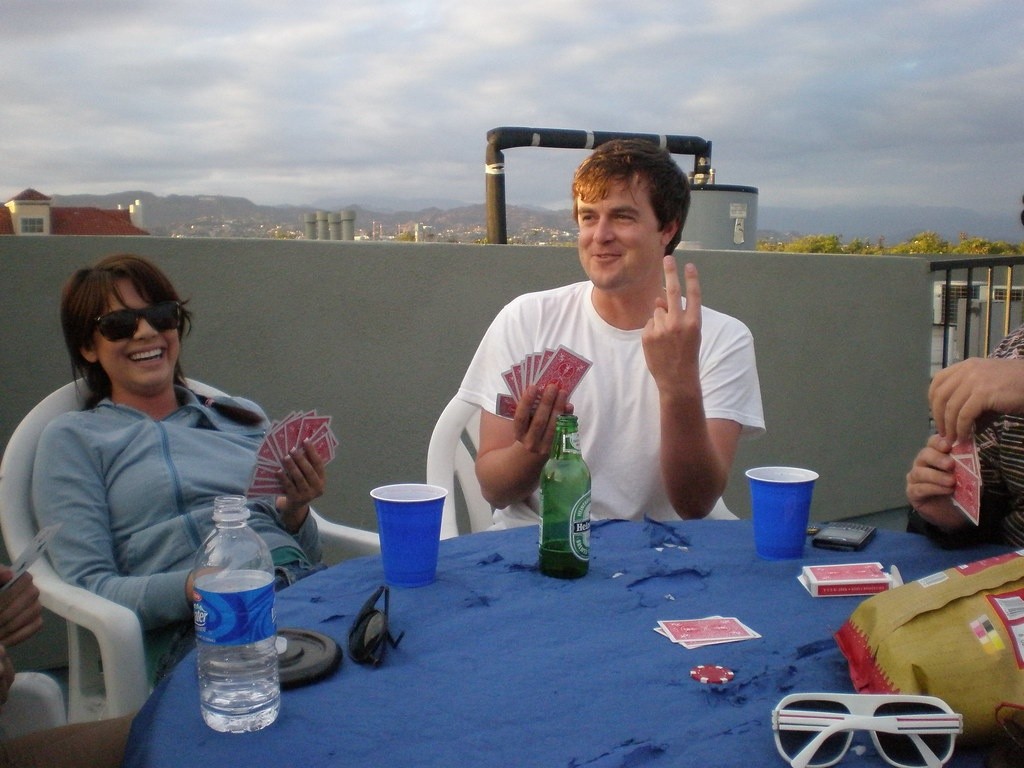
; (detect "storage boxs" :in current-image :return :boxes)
[802,562,903,598]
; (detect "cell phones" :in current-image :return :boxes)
[812,522,877,552]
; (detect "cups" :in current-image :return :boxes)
[746,467,819,561]
[370,483,447,588]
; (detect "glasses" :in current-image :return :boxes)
[94,301,179,341]
[347,586,405,667]
[771,693,963,768]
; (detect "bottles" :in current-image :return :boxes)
[539,414,592,578]
[192,495,280,734]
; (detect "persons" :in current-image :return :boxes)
[457,138,766,531]
[0,564,139,768]
[906,194,1024,552]
[32,254,327,694]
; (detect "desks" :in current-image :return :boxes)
[121,520,1024,768]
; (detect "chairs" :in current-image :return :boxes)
[427,393,740,541]
[0,375,381,723]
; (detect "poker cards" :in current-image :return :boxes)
[0,521,65,594]
[653,615,762,650]
[496,344,594,420]
[244,408,340,499]
[943,424,983,527]
